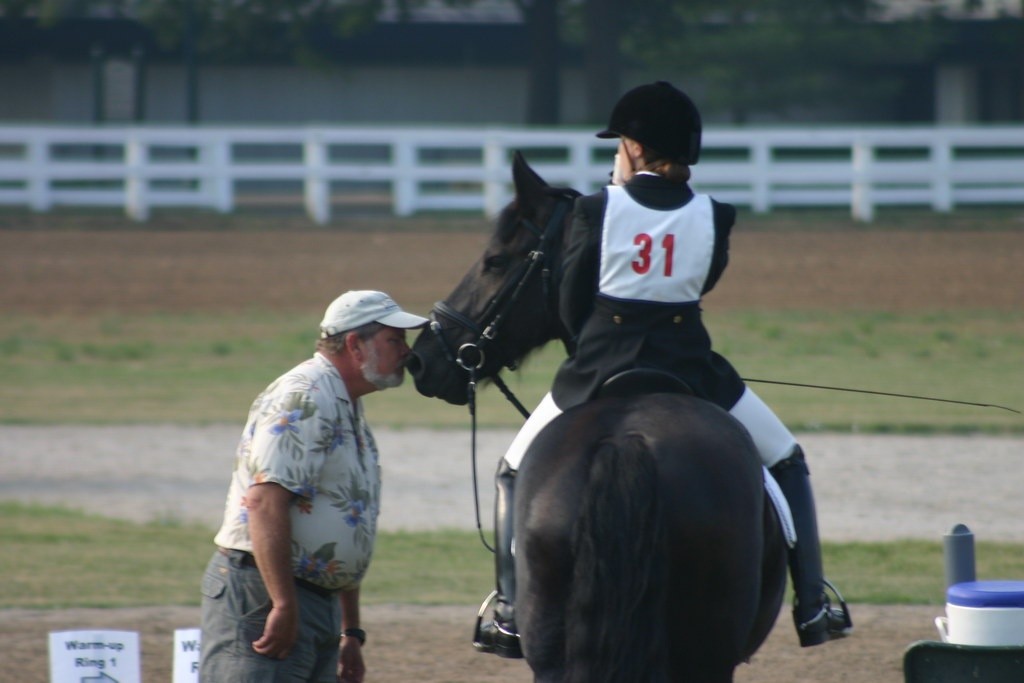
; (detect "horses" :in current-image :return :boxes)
[408,150,789,682]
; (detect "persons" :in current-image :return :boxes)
[473,84,853,659]
[198,290,432,683]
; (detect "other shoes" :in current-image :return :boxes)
[793,603,847,647]
[479,611,522,658]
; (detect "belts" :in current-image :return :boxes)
[217,545,332,598]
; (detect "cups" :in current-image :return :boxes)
[612,155,626,185]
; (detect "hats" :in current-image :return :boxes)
[595,82,702,163]
[319,289,430,339]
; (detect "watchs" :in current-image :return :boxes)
[340,628,366,646]
